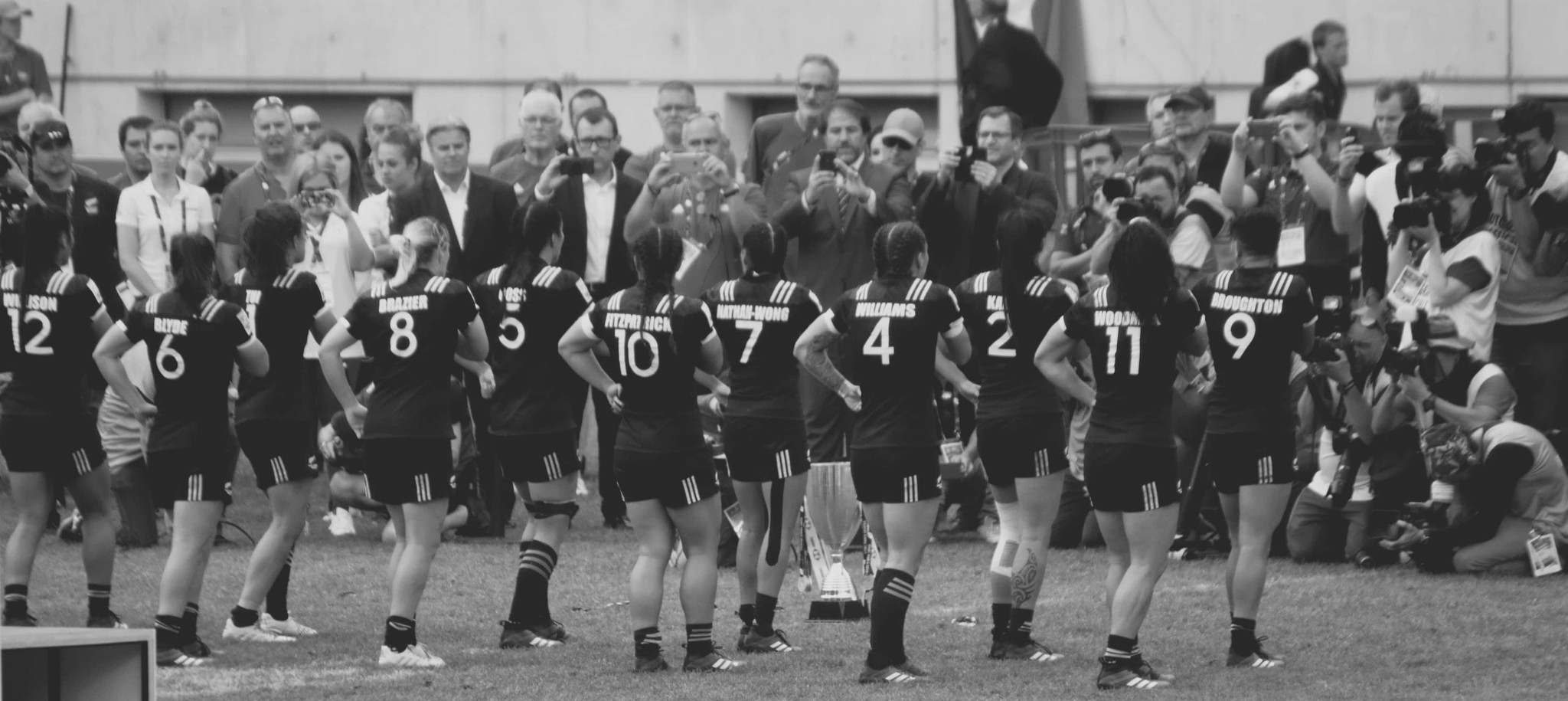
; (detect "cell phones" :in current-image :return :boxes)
[1248,119,1279,137]
[668,153,707,173]
[1345,127,1361,146]
[560,158,594,176]
[301,190,333,208]
[955,147,987,182]
[819,149,837,177]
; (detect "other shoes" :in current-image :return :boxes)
[13,468,629,546]
[3,614,37,628]
[87,610,128,629]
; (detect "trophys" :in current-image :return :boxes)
[797,456,874,622]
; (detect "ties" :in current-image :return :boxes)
[837,186,850,232]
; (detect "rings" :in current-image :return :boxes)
[982,173,987,179]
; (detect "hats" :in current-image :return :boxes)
[1420,422,1480,481]
[0,0,32,19]
[879,107,926,145]
[1398,312,1477,351]
[30,120,70,150]
[1165,83,1210,110]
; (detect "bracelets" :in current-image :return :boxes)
[1292,145,1312,159]
[647,184,662,197]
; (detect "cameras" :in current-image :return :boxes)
[1393,343,1436,384]
[1474,133,1525,169]
[1393,194,1453,229]
[1116,197,1161,225]
[1102,175,1135,202]
[1301,333,1354,364]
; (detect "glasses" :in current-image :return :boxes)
[1079,127,1114,142]
[881,135,913,152]
[35,137,69,151]
[796,80,834,93]
[575,131,615,149]
[293,121,321,133]
[1137,138,1177,165]
[253,96,284,112]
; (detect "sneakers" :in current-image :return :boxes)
[535,620,577,643]
[377,641,444,670]
[221,619,297,645]
[627,501,1479,691]
[406,639,437,659]
[156,649,211,666]
[257,610,319,636]
[182,634,224,657]
[498,618,561,647]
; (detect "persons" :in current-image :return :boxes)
[792,219,973,682]
[1192,207,1319,671]
[0,0,1567,628]
[934,208,1092,660]
[695,219,824,657]
[216,200,338,645]
[317,217,489,671]
[558,224,748,673]
[1032,221,1208,691]
[452,200,595,648]
[94,230,271,659]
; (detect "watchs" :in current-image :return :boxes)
[1422,394,1439,413]
[1420,531,1436,547]
[724,189,742,198]
[1508,186,1532,200]
[1335,378,1351,393]
